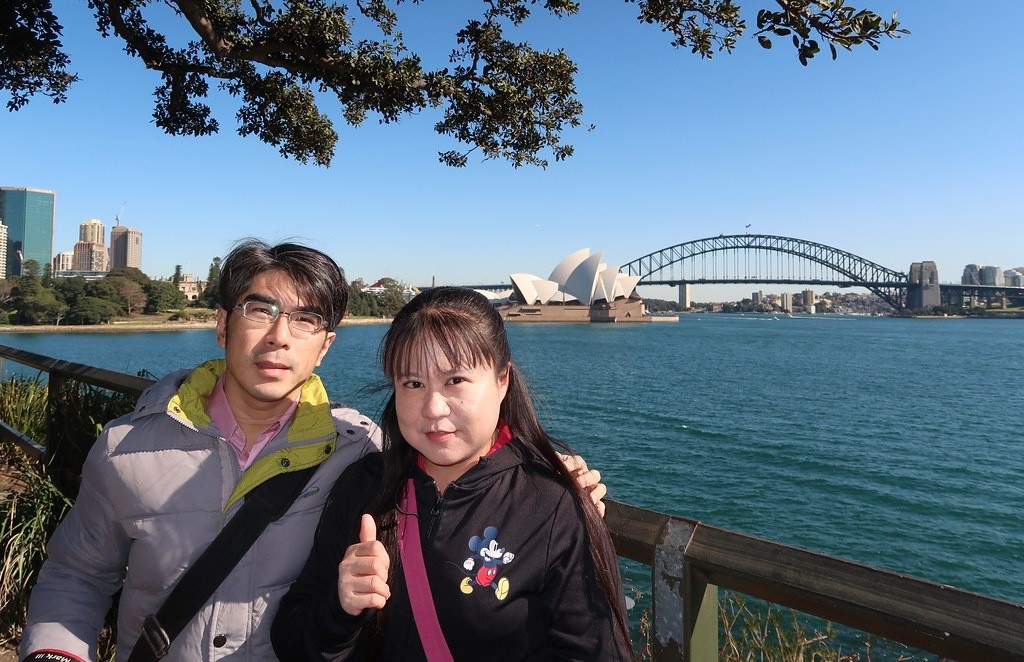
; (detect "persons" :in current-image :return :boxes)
[270,286,633,662]
[20,236,606,661]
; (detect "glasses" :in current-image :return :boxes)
[229,300,330,333]
